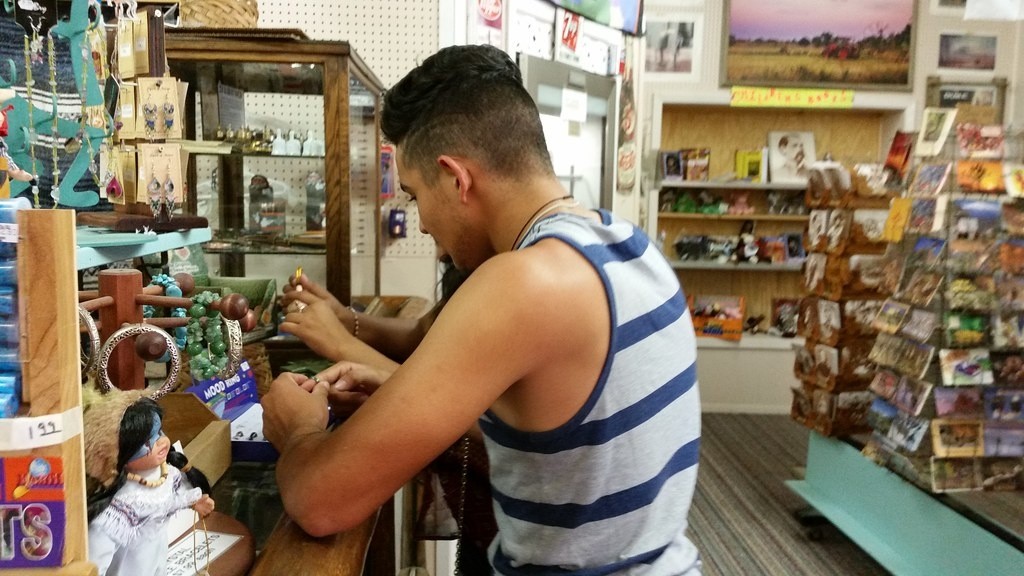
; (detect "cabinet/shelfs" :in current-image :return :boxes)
[0,0,1024,576]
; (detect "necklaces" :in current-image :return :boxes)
[24,30,60,209]
[79,23,113,186]
[517,201,578,243]
[126,462,168,487]
[510,195,574,250]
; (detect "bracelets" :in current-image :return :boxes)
[97,323,180,401]
[141,274,243,381]
[346,307,359,335]
[78,305,100,377]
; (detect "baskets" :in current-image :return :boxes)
[179,0,258,28]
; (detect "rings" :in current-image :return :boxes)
[295,301,307,312]
[310,376,319,382]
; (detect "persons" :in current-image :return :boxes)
[83,389,214,576]
[261,44,703,576]
[0,79,37,198]
